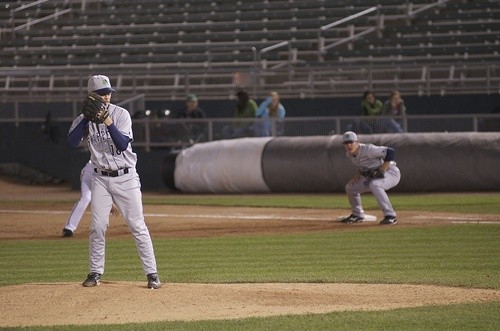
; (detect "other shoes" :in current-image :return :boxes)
[342,214,365,224]
[63,228,73,236]
[379,215,398,225]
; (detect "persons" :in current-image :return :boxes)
[341,131,401,223]
[375,90,408,133]
[252,91,286,137]
[66,74,161,289]
[174,93,213,150]
[351,90,385,135]
[62,159,121,237]
[227,89,258,139]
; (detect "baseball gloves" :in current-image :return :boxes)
[83,96,110,124]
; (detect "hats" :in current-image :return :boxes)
[87,75,116,96]
[342,131,358,144]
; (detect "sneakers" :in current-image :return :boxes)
[146,273,160,289]
[82,272,100,287]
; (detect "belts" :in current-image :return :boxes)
[93,168,128,177]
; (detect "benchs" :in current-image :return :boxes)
[0,1,500,99]
